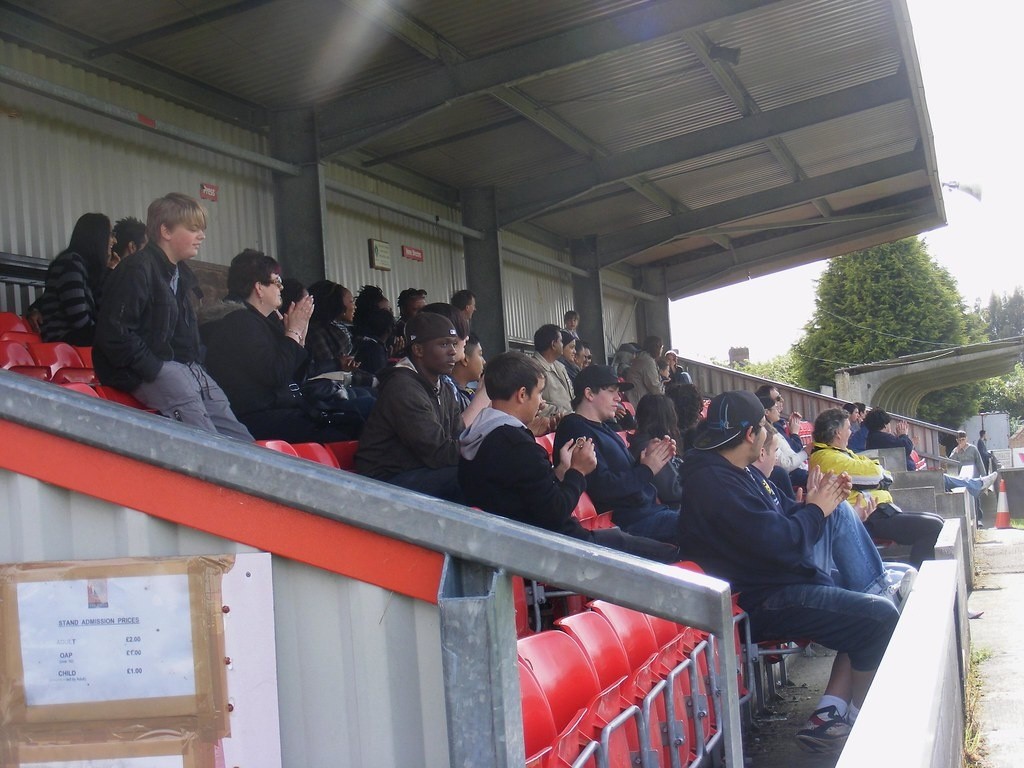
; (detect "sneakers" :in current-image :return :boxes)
[794,705,852,756]
[978,520,983,526]
[876,568,918,615]
[974,471,998,499]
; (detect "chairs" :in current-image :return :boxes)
[0,313,927,768]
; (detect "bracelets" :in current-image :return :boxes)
[285,328,302,340]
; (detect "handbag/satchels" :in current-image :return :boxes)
[316,409,362,434]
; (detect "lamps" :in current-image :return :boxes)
[709,43,742,66]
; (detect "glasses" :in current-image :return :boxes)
[255,276,283,287]
[110,231,117,237]
[771,394,782,401]
[585,354,592,359]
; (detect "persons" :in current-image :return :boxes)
[23,191,947,608]
[977,430,994,476]
[678,389,900,758]
[863,406,998,499]
[948,430,987,530]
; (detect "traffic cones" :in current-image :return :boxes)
[995,479,1011,530]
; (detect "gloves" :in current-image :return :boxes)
[876,476,893,491]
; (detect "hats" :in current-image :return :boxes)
[862,407,892,431]
[619,343,642,352]
[391,312,458,358]
[560,330,575,346]
[693,389,764,450]
[574,364,635,395]
